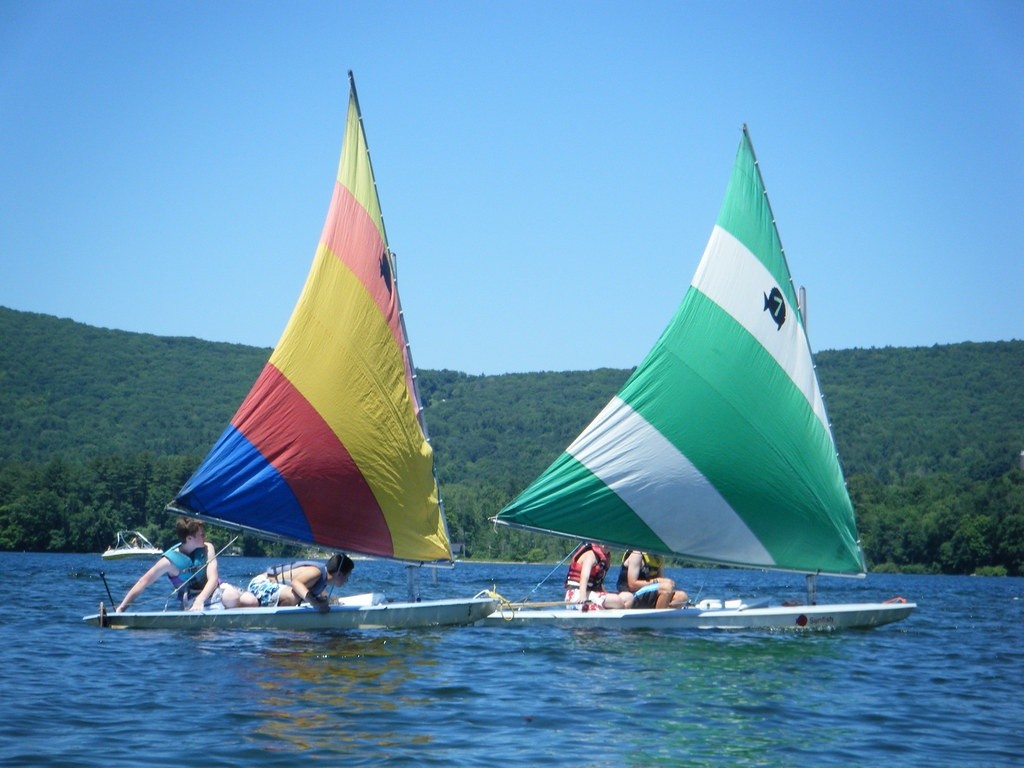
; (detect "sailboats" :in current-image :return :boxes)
[491,121,920,634]
[83,65,508,636]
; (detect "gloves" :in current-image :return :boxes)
[304,590,330,615]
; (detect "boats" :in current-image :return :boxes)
[101,530,163,560]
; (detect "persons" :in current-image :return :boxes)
[129,535,140,549]
[564,542,634,610]
[115,515,258,612]
[249,553,354,613]
[616,549,688,608]
